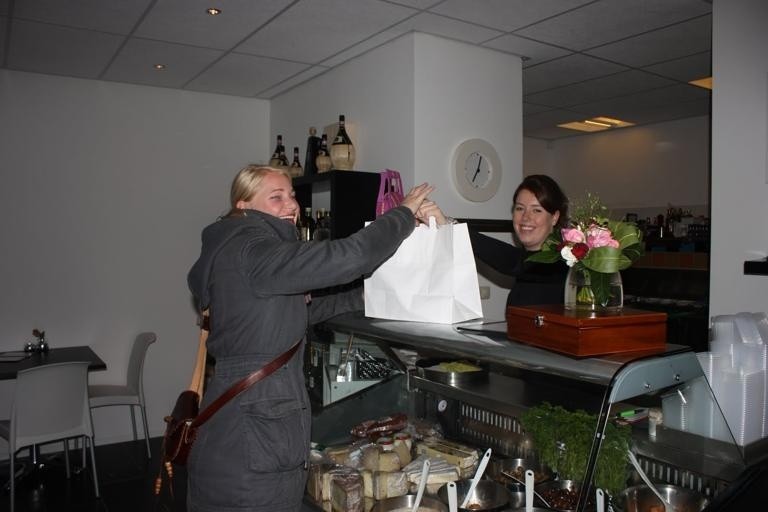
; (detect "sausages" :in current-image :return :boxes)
[350,411,407,438]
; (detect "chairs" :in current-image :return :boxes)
[73,331,161,472]
[0,359,105,512]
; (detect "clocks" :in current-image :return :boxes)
[451,134,501,204]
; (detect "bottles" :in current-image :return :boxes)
[295,207,331,244]
[624,202,711,250]
[35,337,51,353]
[24,341,36,351]
[265,113,356,174]
[376,437,394,451]
[648,406,663,436]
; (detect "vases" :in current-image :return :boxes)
[562,262,630,318]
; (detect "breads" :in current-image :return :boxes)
[306,434,481,512]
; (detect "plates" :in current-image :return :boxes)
[623,296,705,310]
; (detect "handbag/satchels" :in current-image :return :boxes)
[158,390,201,467]
[363,211,484,327]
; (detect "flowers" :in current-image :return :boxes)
[528,190,653,272]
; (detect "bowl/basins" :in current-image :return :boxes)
[434,458,603,511]
[414,357,490,383]
[369,494,448,512]
[610,482,711,512]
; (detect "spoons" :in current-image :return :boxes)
[627,450,678,512]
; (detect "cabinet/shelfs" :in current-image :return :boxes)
[301,307,751,512]
[282,170,382,241]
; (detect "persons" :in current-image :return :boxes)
[418,176,571,319]
[187,165,434,512]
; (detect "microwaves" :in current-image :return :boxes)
[393,433,414,452]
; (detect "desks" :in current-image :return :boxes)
[0,345,112,490]
